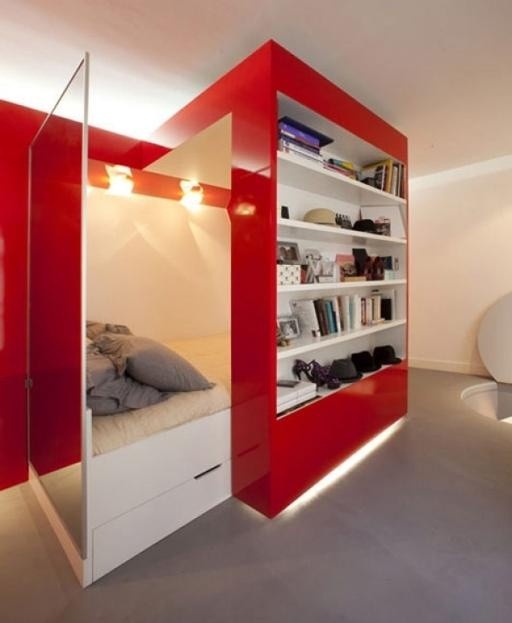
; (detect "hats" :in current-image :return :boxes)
[330,360,362,383]
[353,220,382,234]
[304,208,336,224]
[352,352,381,371]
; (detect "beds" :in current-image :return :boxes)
[88,333,231,583]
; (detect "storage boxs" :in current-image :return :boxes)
[277,264,301,287]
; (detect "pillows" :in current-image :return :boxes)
[89,320,217,416]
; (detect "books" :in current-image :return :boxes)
[277,378,321,418]
[290,288,395,337]
[277,115,404,198]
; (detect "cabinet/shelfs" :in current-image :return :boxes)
[272,39,407,511]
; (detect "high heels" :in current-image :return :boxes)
[312,362,339,388]
[294,360,314,381]
[374,346,400,364]
